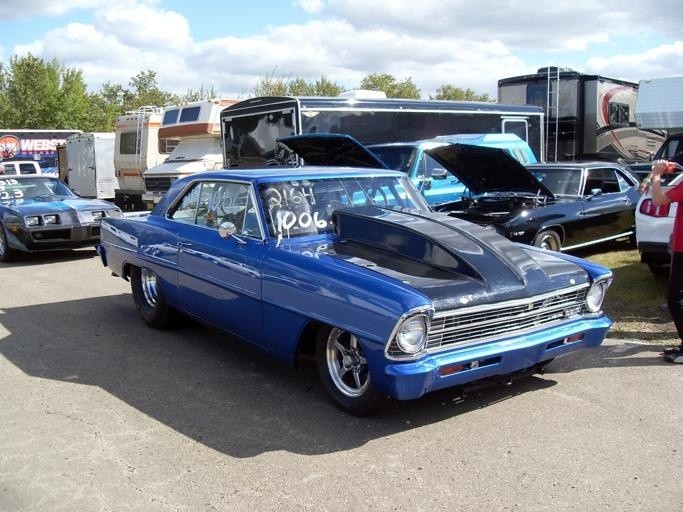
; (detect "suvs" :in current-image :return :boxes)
[0,160,41,175]
[222,63,638,169]
[624,132,682,180]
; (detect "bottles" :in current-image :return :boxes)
[637,171,654,195]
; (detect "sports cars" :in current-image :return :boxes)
[96,166,615,420]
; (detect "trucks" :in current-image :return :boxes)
[66,131,146,211]
[144,89,390,210]
[114,105,171,211]
[275,132,538,221]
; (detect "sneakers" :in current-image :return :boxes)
[663,353,683,365]
[663,344,683,355]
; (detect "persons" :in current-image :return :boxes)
[652,159,683,364]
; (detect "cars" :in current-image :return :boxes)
[422,141,643,256]
[634,173,682,278]
[55,143,68,181]
[1,174,122,259]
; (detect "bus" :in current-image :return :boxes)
[0,129,85,175]
